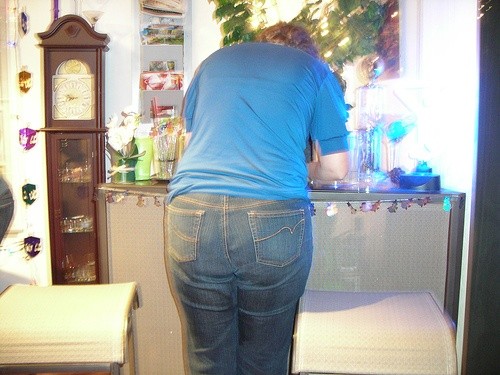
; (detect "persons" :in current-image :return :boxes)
[164,23,351,375]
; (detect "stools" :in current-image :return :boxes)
[0,279,144,375]
[289,290,460,375]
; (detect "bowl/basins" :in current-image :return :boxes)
[399,171,440,190]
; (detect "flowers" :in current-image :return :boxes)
[104,115,179,147]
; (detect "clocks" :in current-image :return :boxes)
[35,14,112,283]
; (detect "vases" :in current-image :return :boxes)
[112,132,182,183]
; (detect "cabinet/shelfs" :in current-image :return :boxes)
[134,0,193,123]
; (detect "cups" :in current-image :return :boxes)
[134,136,154,180]
[60,215,94,233]
[153,127,178,180]
[61,253,96,282]
[57,159,91,184]
